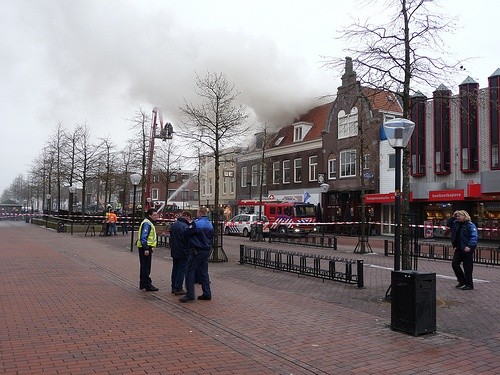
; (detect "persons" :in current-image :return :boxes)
[180,206,214,301]
[136,208,159,290]
[170,212,192,295]
[446,210,478,290]
[121,209,128,235]
[105,210,117,235]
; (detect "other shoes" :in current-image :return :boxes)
[456,282,464,288]
[179,296,194,301]
[461,286,473,290]
[197,294,211,300]
[146,286,158,291]
[172,286,176,293]
[140,283,146,289]
[175,290,186,295]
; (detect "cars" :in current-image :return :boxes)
[224,214,270,237]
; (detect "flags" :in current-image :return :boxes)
[304,192,311,202]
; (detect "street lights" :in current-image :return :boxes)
[382,118,418,300]
[129,173,143,252]
[45,193,51,228]
[69,186,75,235]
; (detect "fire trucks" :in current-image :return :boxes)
[153,202,178,222]
[238,199,318,236]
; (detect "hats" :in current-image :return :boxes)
[182,212,195,221]
